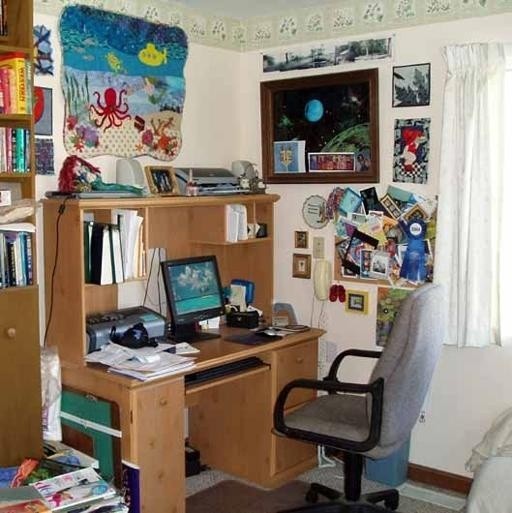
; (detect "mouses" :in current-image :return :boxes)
[254,329,277,337]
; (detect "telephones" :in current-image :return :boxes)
[313,261,331,300]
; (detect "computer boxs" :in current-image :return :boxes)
[86,306,169,354]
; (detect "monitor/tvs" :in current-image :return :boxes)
[159,254,226,344]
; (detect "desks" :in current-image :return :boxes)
[41,193,327,512]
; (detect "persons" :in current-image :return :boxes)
[152,172,171,193]
[357,154,370,171]
[59,155,149,197]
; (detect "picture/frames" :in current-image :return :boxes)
[291,230,311,279]
[260,68,380,184]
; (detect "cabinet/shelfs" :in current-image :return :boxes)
[1,0,40,466]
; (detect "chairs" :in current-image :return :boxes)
[270,281,446,512]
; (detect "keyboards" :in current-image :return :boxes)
[184,357,264,388]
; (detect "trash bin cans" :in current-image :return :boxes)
[364,430,409,486]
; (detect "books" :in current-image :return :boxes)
[107,359,195,381]
[0,50,36,287]
[0,467,117,513]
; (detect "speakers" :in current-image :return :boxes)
[115,158,145,187]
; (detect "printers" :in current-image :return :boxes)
[174,167,242,196]
